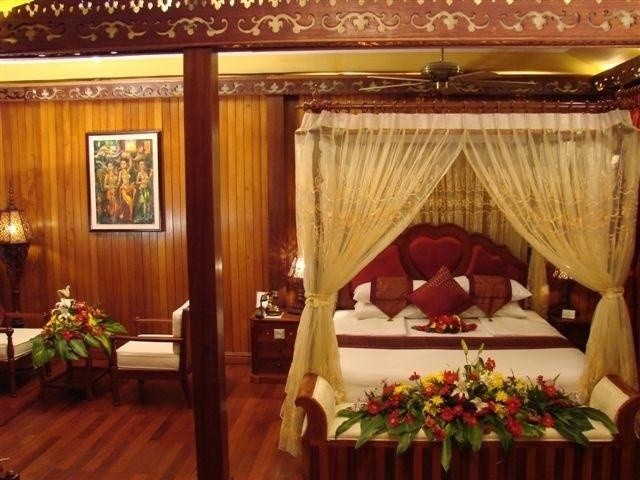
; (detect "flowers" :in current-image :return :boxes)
[29,282,131,369]
[408,312,479,335]
[332,337,622,472]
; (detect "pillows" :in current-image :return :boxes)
[352,278,428,303]
[352,300,426,321]
[405,264,477,321]
[454,271,534,302]
[461,302,530,319]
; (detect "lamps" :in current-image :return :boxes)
[287,256,306,315]
[552,266,579,316]
[0,179,38,328]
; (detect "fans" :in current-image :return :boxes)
[358,49,540,96]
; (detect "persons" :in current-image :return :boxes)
[103,161,153,222]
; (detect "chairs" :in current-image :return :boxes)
[0,302,52,398]
[110,297,193,411]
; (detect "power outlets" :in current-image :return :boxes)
[270,291,278,298]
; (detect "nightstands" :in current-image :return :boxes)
[249,305,304,375]
[548,310,594,354]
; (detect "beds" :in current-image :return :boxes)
[277,79,638,464]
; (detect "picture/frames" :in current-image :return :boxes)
[84,130,164,233]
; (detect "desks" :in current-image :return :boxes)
[33,339,112,400]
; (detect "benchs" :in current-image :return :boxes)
[294,367,639,478]
